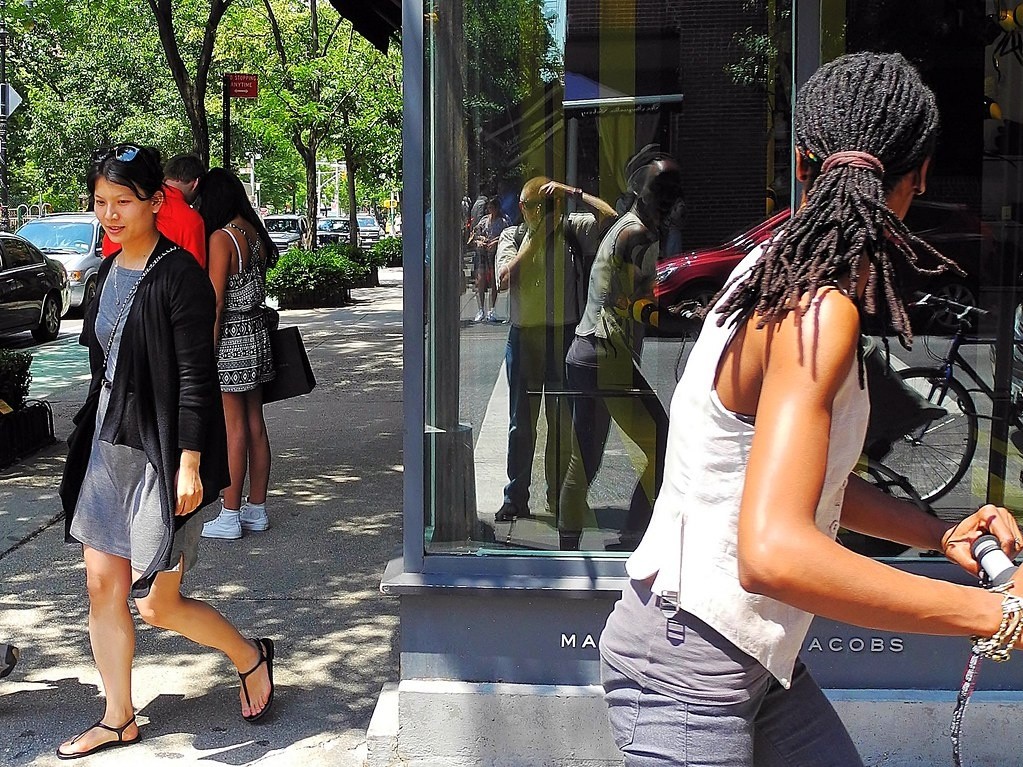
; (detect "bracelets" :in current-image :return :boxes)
[572,188,582,199]
[968,580,1023,663]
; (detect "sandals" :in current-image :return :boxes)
[56,714,142,760]
[238,638,275,721]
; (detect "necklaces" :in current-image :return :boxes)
[119,247,153,305]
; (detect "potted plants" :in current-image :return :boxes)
[0,348,50,472]
[266,235,403,310]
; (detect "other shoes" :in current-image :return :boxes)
[494,503,531,524]
[0,643,19,678]
[557,530,579,548]
[475,312,485,322]
[545,498,597,525]
[486,307,496,321]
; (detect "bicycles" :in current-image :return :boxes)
[862,290,1023,506]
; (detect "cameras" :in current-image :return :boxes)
[540,195,553,216]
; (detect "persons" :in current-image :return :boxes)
[557,153,699,550]
[101,153,279,540]
[597,51,970,766]
[462,196,509,322]
[496,175,617,524]
[57,143,272,758]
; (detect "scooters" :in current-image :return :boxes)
[378,218,386,232]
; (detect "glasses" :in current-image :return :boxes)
[519,197,562,211]
[91,145,152,168]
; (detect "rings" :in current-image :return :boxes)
[1015,538,1020,542]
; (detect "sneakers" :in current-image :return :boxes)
[200,498,242,539]
[239,495,269,530]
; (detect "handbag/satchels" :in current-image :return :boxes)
[474,236,492,253]
[259,326,316,404]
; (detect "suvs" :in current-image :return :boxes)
[315,216,363,251]
[356,212,386,251]
[263,214,309,257]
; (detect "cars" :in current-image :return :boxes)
[650,200,1022,338]
[13,211,105,319]
[0,231,72,345]
